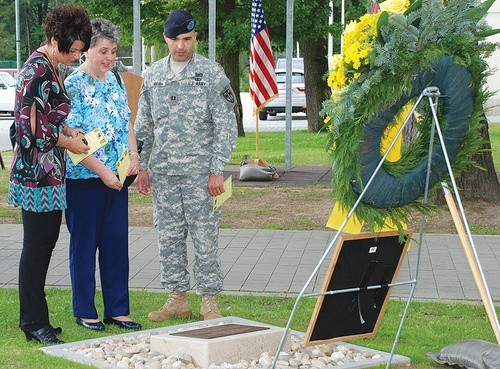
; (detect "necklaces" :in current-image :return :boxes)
[43,45,59,76]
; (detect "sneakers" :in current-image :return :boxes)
[148,292,224,321]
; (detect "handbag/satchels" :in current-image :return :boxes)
[9,120,16,152]
[128,139,144,185]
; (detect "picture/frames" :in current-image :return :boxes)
[304,229,413,347]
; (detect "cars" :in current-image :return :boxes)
[0,72,17,116]
[259,68,306,120]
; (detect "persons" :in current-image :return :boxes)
[134,10,238,321]
[64,18,141,330]
[8,4,90,345]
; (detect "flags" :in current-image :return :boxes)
[249,0,279,116]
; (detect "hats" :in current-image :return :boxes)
[164,10,196,37]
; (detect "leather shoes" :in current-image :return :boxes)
[77,315,142,332]
[23,325,65,345]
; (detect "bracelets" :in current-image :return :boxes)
[59,137,72,148]
[129,149,139,158]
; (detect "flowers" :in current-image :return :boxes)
[323,0,410,131]
